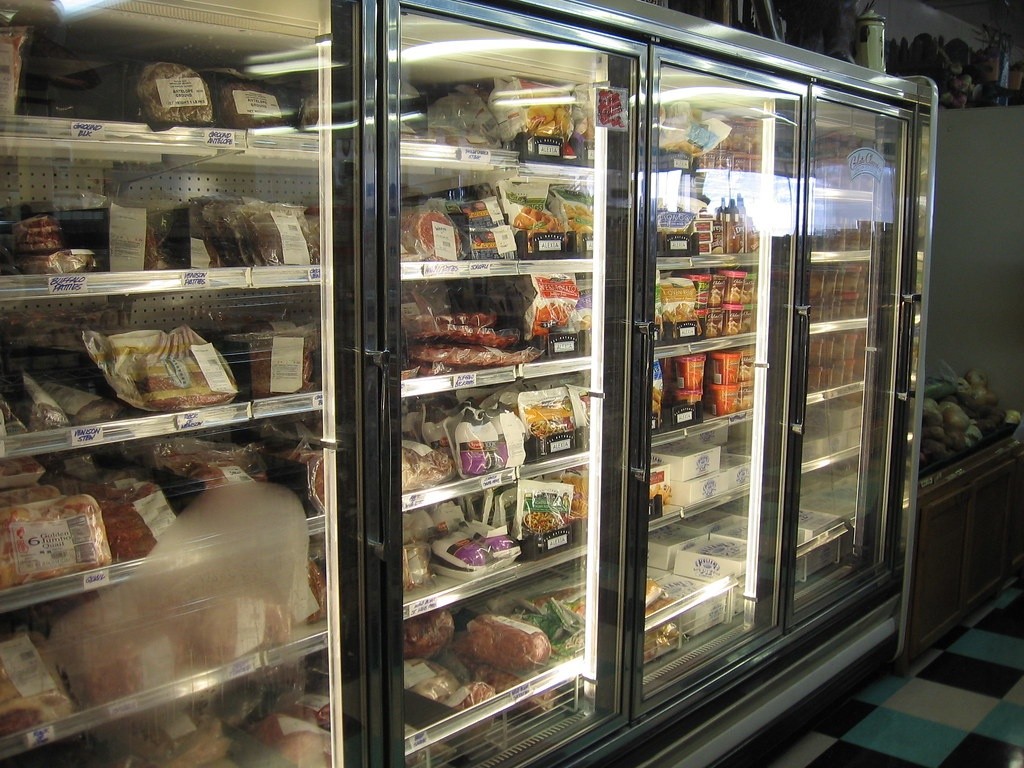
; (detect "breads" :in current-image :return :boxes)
[513,203,595,252]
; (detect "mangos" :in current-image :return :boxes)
[527,105,574,143]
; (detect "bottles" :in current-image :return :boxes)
[717,193,749,253]
[858,9,887,73]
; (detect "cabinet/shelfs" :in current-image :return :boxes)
[894,438,1024,677]
